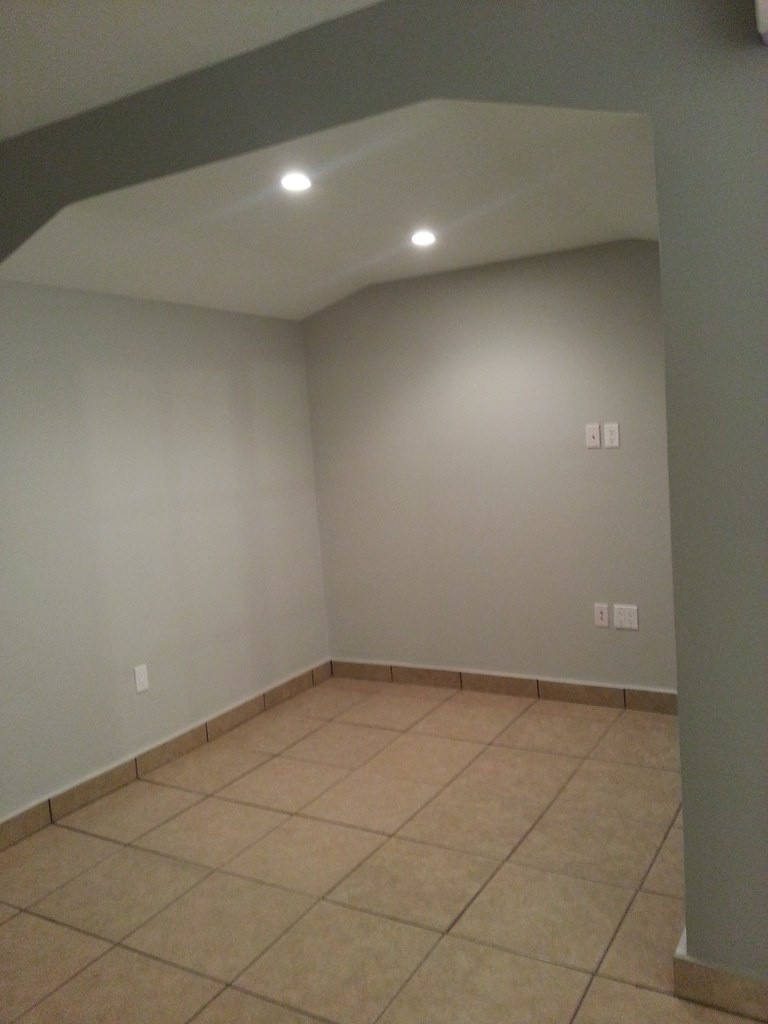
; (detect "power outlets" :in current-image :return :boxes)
[604,423,619,448]
[613,605,639,629]
[594,604,608,627]
[585,423,600,449]
[135,664,149,692]
